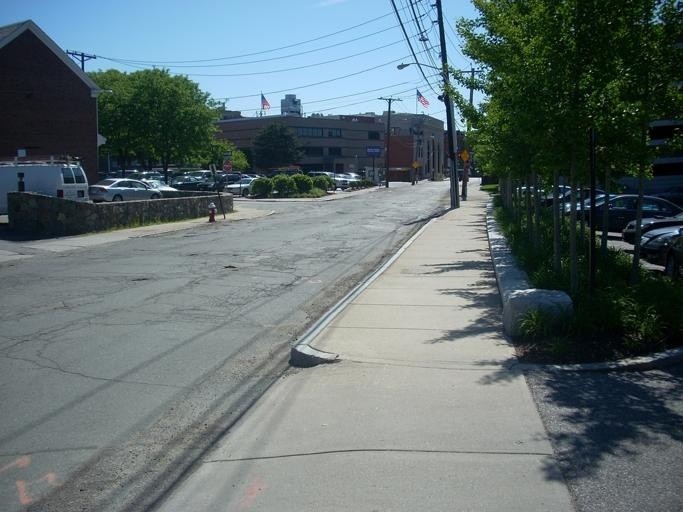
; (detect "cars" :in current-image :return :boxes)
[337,172,362,187]
[516,185,683,280]
[89,168,270,202]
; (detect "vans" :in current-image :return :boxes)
[0,156,91,215]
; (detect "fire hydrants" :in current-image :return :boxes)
[207,202,217,222]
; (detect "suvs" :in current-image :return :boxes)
[306,172,350,191]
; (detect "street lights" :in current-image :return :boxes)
[397,62,483,201]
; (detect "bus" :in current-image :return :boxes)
[269,168,303,178]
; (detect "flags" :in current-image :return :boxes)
[417,89,430,109]
[261,93,270,110]
